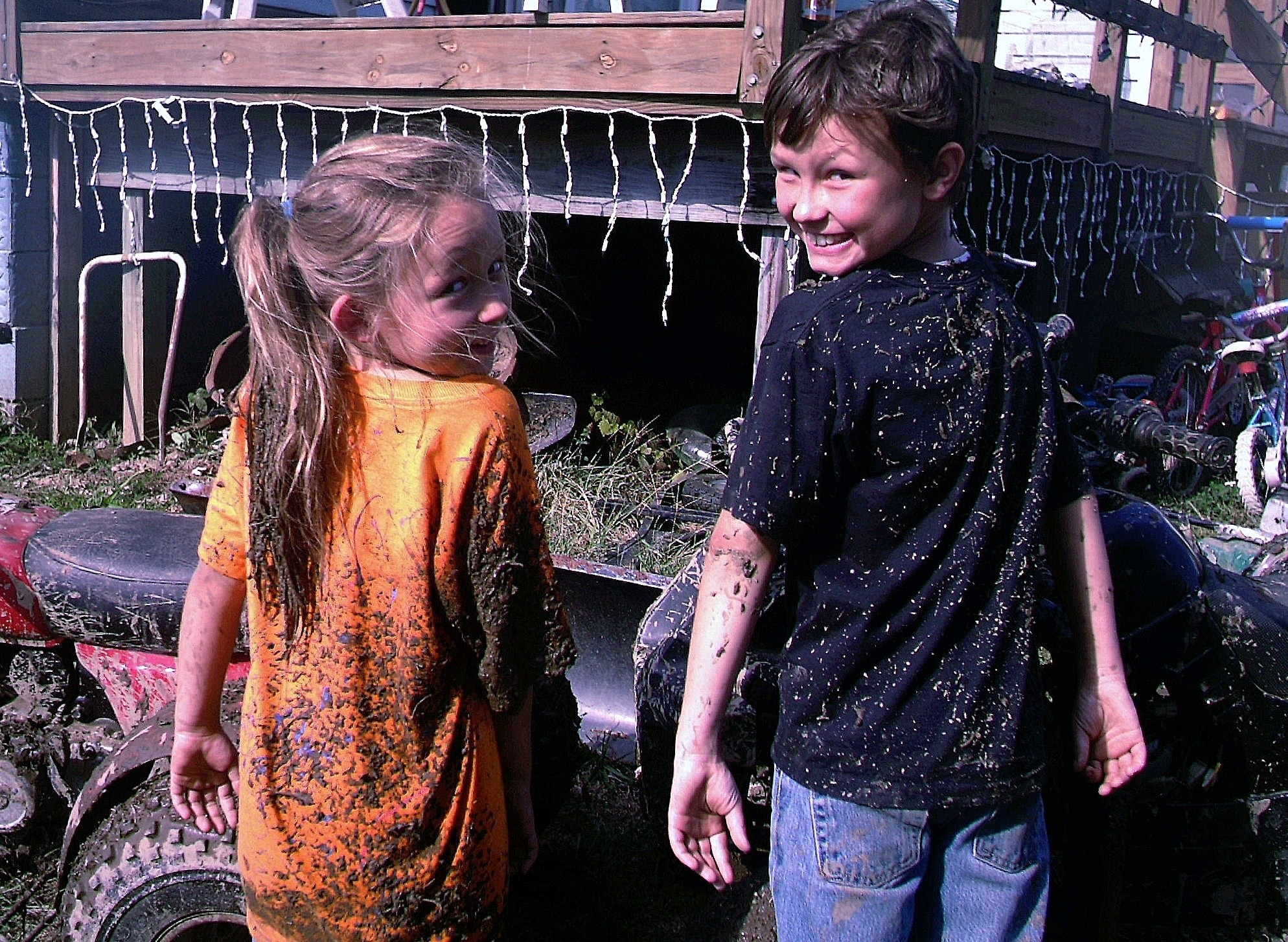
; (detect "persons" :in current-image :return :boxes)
[167,131,579,942]
[665,0,1147,940]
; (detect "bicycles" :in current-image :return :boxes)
[983,206,1288,517]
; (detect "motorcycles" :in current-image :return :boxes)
[0,383,1288,940]
